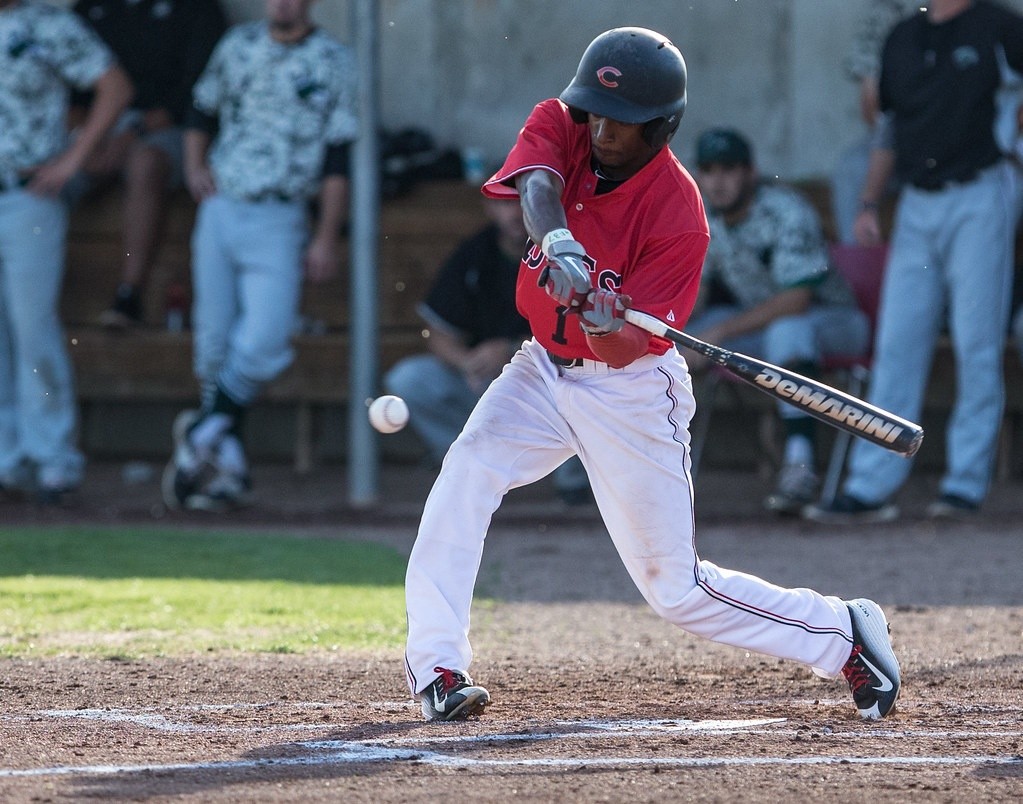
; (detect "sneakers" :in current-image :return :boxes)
[420,665,490,721]
[842,595,902,721]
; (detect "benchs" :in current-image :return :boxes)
[63,172,832,478]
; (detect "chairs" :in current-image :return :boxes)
[689,243,1023,522]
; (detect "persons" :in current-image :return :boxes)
[71,0,228,330]
[0,0,133,506]
[402,26,901,721]
[678,126,872,512]
[801,0,1023,524]
[382,159,593,502]
[162,0,361,513]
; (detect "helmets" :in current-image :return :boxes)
[560,27,687,150]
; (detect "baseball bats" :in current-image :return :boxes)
[538,268,928,458]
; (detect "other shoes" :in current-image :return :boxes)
[21,481,77,515]
[185,477,255,515]
[763,465,816,513]
[97,286,145,331]
[929,490,975,516]
[158,408,206,510]
[805,490,896,526]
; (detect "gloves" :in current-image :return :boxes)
[577,284,632,339]
[539,225,593,310]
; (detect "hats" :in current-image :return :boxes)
[696,127,751,169]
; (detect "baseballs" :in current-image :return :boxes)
[367,394,410,435]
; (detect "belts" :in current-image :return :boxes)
[547,346,585,369]
